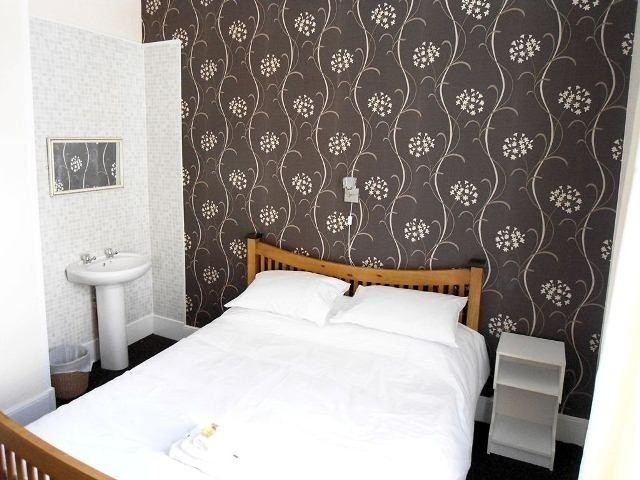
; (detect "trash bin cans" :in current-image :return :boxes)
[50,343,90,400]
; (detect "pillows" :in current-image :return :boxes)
[330,284,468,349]
[223,270,352,326]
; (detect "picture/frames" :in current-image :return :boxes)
[45,134,125,197]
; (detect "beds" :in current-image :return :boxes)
[0,233,491,480]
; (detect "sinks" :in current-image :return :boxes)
[66,254,152,286]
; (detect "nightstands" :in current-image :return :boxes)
[487,332,567,472]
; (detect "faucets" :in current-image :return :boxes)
[104,248,118,258]
[81,255,96,264]
